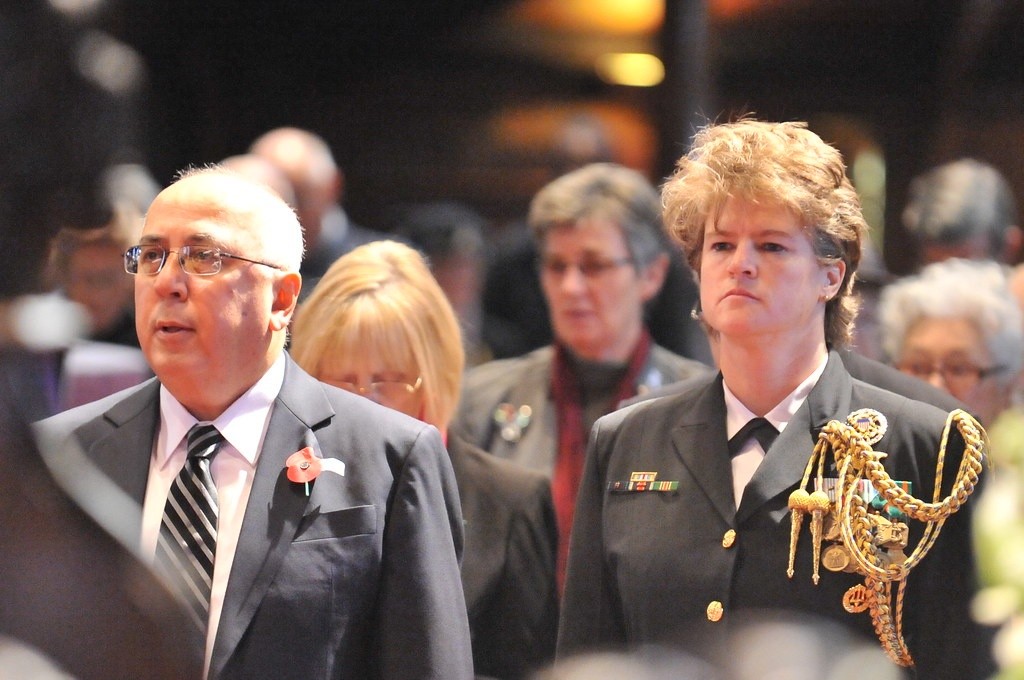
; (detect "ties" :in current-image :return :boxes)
[154,424,225,680]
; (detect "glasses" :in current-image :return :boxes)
[320,371,423,409]
[533,253,632,278]
[899,361,1005,397]
[120,246,283,274]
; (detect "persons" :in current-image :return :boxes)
[0,128,488,415]
[557,119,1003,680]
[454,158,1024,601]
[30,165,476,680]
[288,241,560,680]
